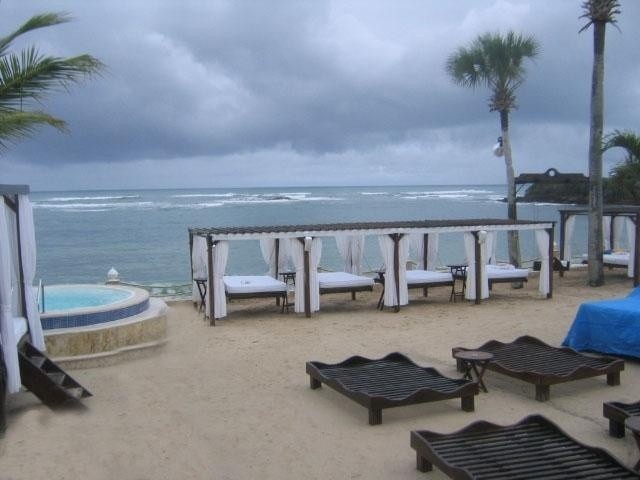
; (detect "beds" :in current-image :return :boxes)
[582,252,629,268]
[488,264,528,291]
[319,272,373,299]
[407,270,453,296]
[224,276,294,306]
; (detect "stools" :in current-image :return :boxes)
[455,351,493,392]
[625,416,640,472]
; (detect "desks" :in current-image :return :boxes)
[279,272,296,313]
[194,277,207,313]
[447,265,468,303]
